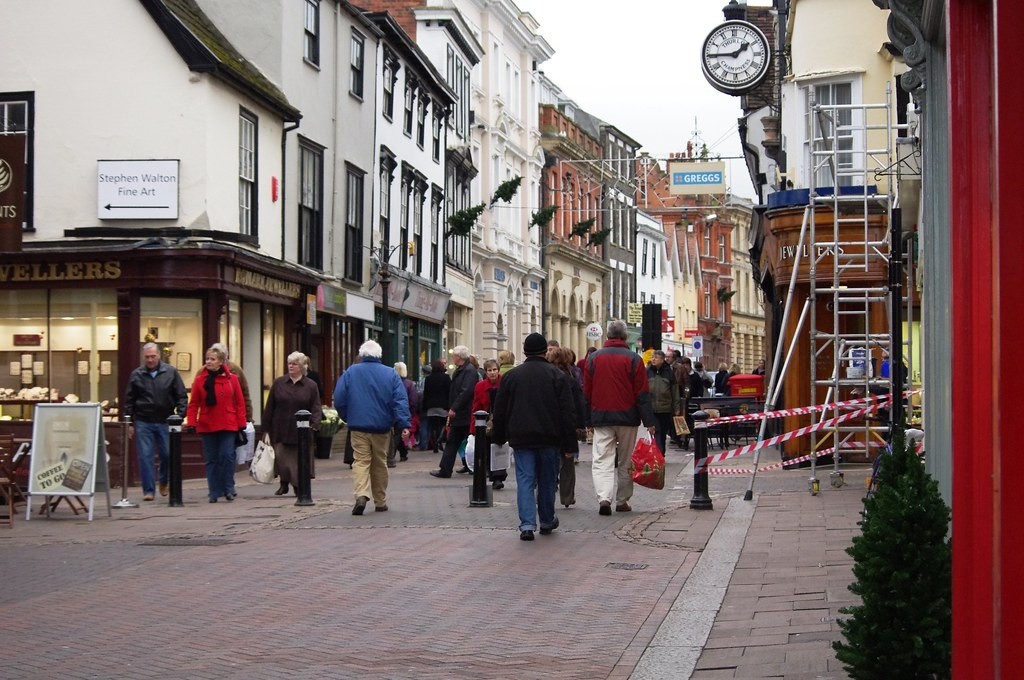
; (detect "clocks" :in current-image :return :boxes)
[701,18,770,95]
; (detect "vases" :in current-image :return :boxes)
[313,436,332,458]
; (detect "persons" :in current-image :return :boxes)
[491,332,578,539]
[333,340,411,515]
[187,343,322,503]
[125,343,189,500]
[583,321,655,515]
[853,342,907,453]
[343,337,767,490]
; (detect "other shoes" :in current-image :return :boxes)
[599,501,611,516]
[352,497,367,513]
[210,496,216,503]
[159,480,168,496]
[275,483,289,495]
[540,518,558,534]
[520,530,535,542]
[376,504,388,512]
[616,504,631,512]
[492,480,505,490]
[225,491,233,500]
[430,469,452,478]
[144,493,154,500]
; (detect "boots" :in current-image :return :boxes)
[456,457,469,474]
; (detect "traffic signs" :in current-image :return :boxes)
[98,159,179,220]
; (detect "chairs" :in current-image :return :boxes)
[704,405,751,450]
[0,433,28,527]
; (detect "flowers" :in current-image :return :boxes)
[315,406,346,436]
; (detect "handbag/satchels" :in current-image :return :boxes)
[674,415,691,436]
[237,431,248,448]
[250,433,275,485]
[466,435,475,473]
[630,431,664,489]
[236,421,255,465]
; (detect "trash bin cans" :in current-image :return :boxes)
[729,374,765,398]
